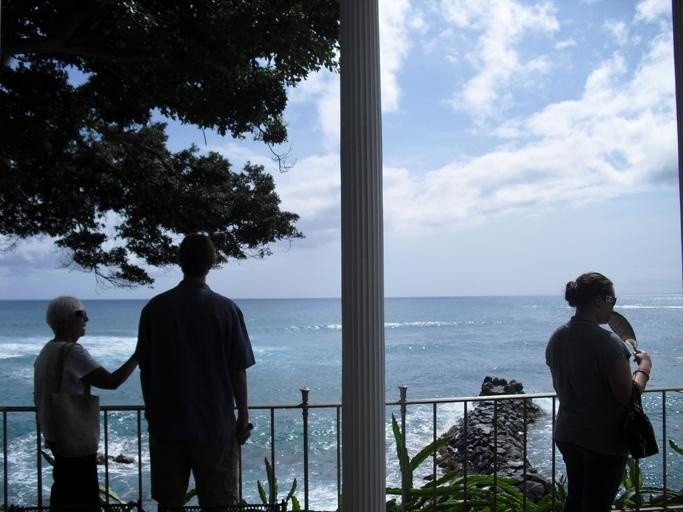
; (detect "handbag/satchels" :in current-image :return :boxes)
[621,380,657,460]
[49,342,100,459]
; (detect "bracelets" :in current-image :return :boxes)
[632,367,650,380]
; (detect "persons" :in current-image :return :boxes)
[32,295,137,512]
[544,273,654,511]
[135,232,256,512]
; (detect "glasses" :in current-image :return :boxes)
[72,310,86,320]
[601,294,616,308]
